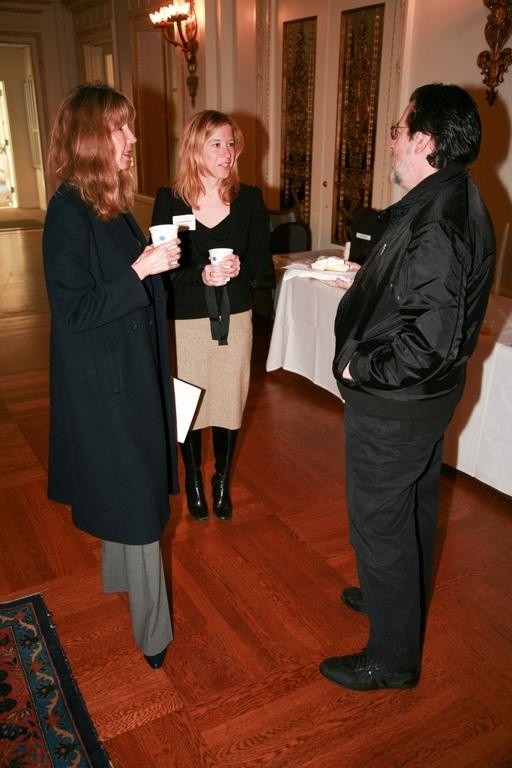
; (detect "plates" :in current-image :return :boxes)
[287,261,362,278]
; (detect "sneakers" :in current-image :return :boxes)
[321,648,419,690]
[341,585,370,615]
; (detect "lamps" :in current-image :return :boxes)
[147,1,200,109]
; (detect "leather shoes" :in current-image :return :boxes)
[144,649,166,669]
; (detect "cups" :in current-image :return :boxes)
[207,246,234,283]
[149,223,180,267]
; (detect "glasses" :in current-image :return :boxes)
[390,121,412,140]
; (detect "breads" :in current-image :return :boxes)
[312,256,349,272]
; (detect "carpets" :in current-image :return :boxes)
[2,592,119,766]
[0,207,44,231]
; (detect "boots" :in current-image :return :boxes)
[211,426,237,520]
[180,429,208,520]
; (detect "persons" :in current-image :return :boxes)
[316,81,498,691]
[148,108,277,522]
[40,81,186,671]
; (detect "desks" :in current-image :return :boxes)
[263,255,512,498]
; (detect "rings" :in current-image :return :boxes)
[210,271,212,276]
